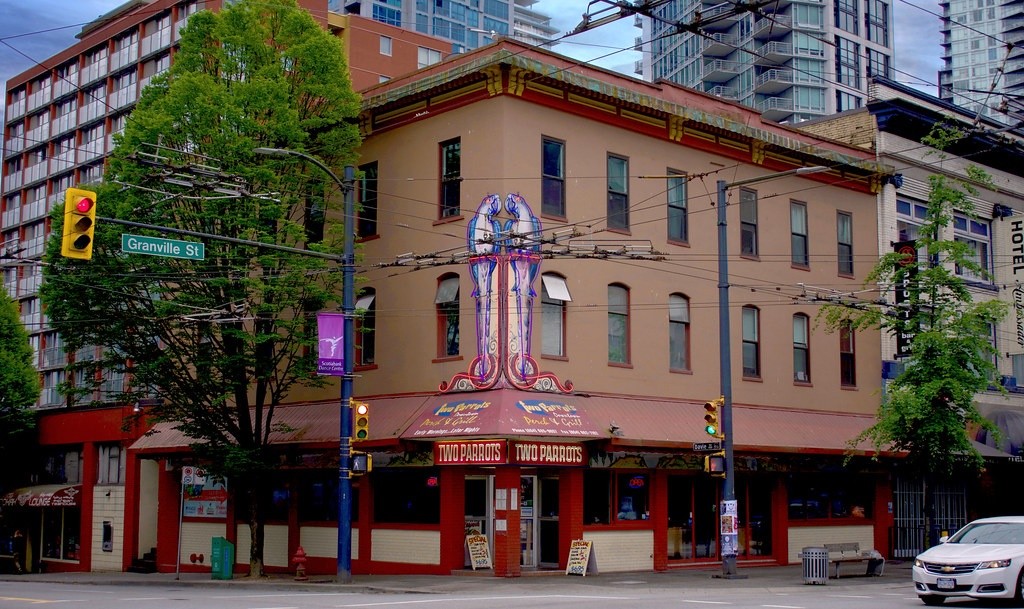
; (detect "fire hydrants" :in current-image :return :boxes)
[292,547,308,581]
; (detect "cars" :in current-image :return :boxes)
[912,516,1024,606]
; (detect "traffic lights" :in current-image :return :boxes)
[61,188,96,260]
[705,401,718,438]
[709,456,726,473]
[351,455,368,471]
[355,404,368,441]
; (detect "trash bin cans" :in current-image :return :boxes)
[798,548,830,585]
[210,536,235,580]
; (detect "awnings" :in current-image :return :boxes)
[0,484,82,507]
[128,396,439,449]
[576,395,1015,457]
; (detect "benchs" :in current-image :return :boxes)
[824,542,886,579]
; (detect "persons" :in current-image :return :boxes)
[9,530,24,573]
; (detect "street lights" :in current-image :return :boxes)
[256,146,356,583]
[716,166,836,576]
